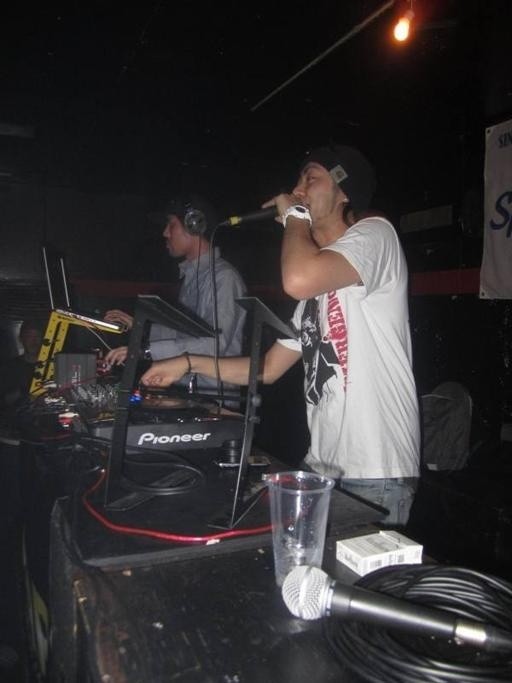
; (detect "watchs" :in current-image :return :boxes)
[282,206,314,228]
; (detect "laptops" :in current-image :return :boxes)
[42,247,128,331]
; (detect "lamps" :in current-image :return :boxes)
[392,9,415,42]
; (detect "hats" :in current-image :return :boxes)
[297,144,378,208]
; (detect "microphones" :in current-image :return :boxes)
[280,564,512,655]
[228,204,280,226]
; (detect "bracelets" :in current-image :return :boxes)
[185,352,191,374]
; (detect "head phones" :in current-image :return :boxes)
[182,197,208,237]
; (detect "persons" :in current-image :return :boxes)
[104,196,246,409]
[16,324,42,361]
[141,146,421,530]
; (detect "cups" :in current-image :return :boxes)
[265,472,335,588]
